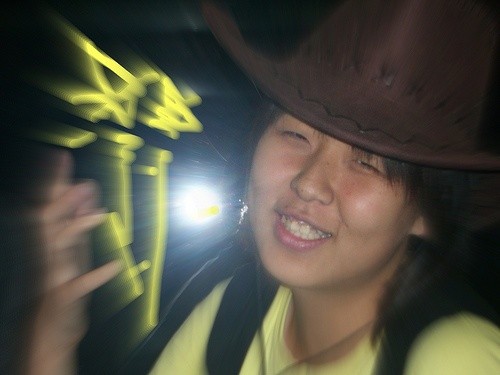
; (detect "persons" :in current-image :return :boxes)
[23,0,500,374]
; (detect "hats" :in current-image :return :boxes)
[201,0,500,171]
